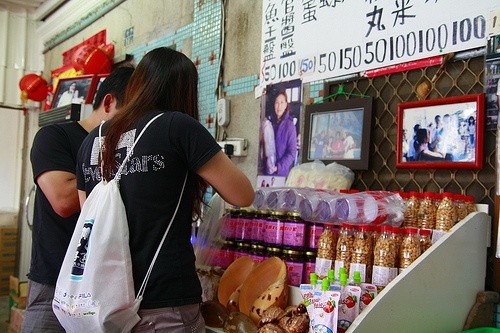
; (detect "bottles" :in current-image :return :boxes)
[314,187,476,296]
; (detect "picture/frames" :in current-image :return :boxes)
[302,96,373,172]
[88,73,110,103]
[395,93,486,171]
[50,74,96,111]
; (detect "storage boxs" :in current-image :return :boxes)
[0,211,29,333]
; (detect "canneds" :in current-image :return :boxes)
[206,208,324,286]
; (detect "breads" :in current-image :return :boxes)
[200,280,310,333]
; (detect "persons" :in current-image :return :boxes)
[57,82,84,108]
[402,113,476,163]
[74,47,256,333]
[261,177,276,187]
[312,130,357,158]
[258,90,298,180]
[20,66,135,333]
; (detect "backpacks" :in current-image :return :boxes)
[51,112,188,333]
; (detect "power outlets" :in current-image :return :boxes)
[217,140,247,158]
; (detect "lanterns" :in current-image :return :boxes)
[20,74,48,104]
[71,43,115,76]
